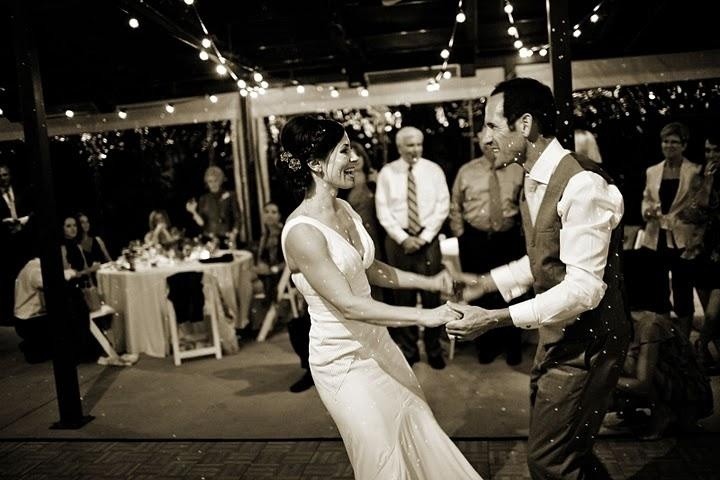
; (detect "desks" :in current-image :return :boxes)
[97,244,255,363]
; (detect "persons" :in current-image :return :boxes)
[442,78,632,480]
[575,124,720,351]
[247,203,285,296]
[145,210,184,248]
[186,166,242,245]
[0,166,32,325]
[342,125,531,369]
[14,258,76,363]
[275,116,484,479]
[287,315,316,394]
[60,213,113,290]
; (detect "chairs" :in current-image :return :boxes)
[257,264,304,346]
[163,270,226,365]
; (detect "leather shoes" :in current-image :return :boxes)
[290,375,314,393]
[480,346,522,365]
[407,353,446,369]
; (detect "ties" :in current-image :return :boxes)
[408,166,420,234]
[489,168,502,232]
